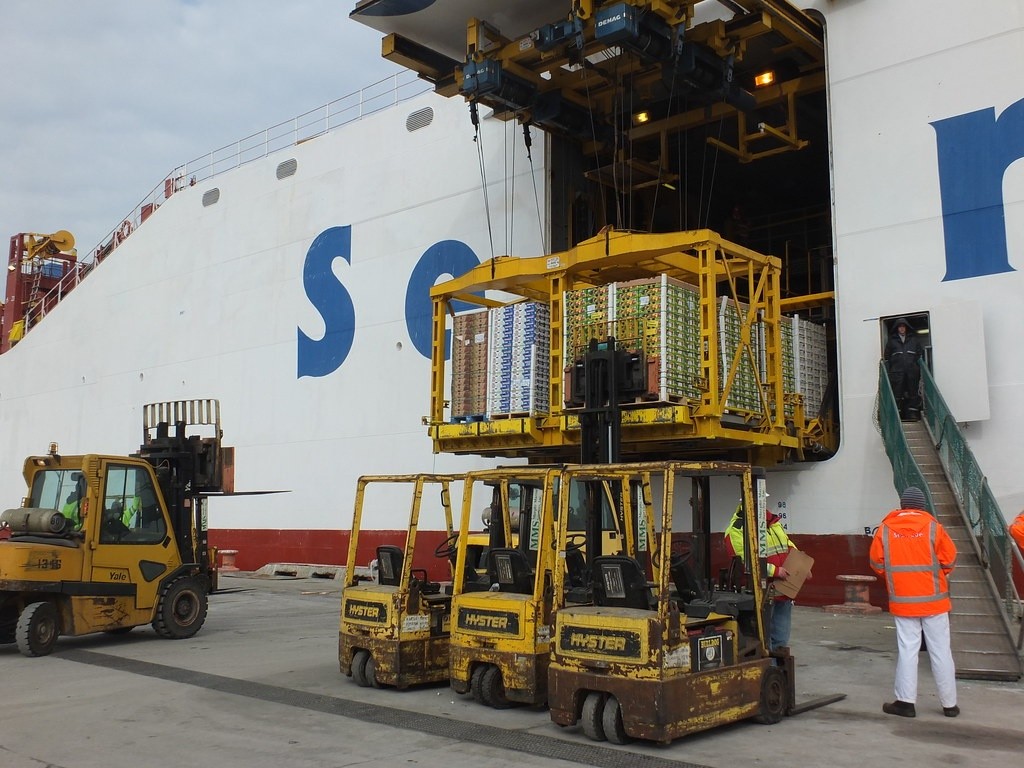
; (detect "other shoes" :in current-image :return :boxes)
[908,413,916,419]
[882,700,916,717]
[943,706,960,717]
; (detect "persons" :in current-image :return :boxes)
[1009,509,1024,553]
[870,486,961,717]
[723,492,812,652]
[884,318,923,421]
[61,475,141,530]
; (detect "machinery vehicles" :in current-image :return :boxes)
[545,452,850,747]
[335,475,524,691]
[446,465,686,712]
[1,400,295,658]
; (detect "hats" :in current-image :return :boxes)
[900,486,926,510]
